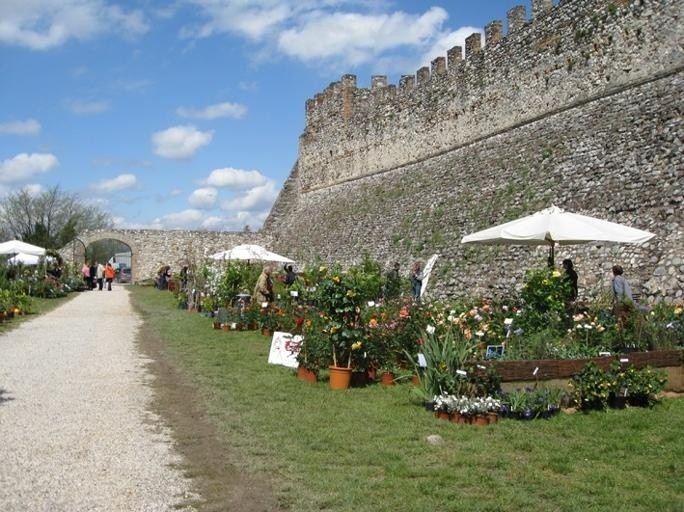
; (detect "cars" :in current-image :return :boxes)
[118,267,132,283]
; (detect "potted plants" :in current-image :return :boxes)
[0,289,17,322]
[212,308,242,331]
[177,290,188,309]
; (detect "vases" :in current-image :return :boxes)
[451,413,498,426]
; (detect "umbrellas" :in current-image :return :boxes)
[210,243,296,270]
[0,238,46,277]
[459,206,657,274]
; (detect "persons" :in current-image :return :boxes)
[254,265,274,305]
[612,265,633,305]
[180,266,189,289]
[281,263,295,288]
[562,260,578,305]
[410,262,427,299]
[152,265,172,290]
[388,262,402,298]
[81,261,127,291]
